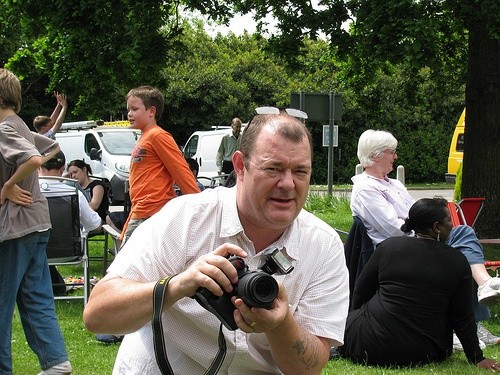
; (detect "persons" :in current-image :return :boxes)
[119,85,202,254]
[0,67,73,374]
[62,157,106,237]
[215,117,243,186]
[35,148,103,296]
[33,90,67,140]
[327,197,500,372]
[105,157,206,250]
[350,129,500,303]
[83,111,350,374]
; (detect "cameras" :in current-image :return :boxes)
[195,247,294,331]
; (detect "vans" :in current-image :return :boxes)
[181,123,249,187]
[53,119,142,206]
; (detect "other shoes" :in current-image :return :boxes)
[96,334,124,342]
[65,281,74,292]
[38,360,72,375]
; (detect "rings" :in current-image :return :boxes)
[491,364,495,367]
[250,322,256,327]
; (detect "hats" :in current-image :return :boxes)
[53,150,65,163]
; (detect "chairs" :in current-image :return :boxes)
[34,176,89,310]
[441,198,500,273]
[126,169,236,222]
[86,176,112,278]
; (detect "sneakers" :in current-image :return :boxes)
[475,323,500,346]
[477,278,500,304]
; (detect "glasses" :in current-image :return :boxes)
[44,123,52,127]
[384,150,396,155]
[243,106,308,141]
